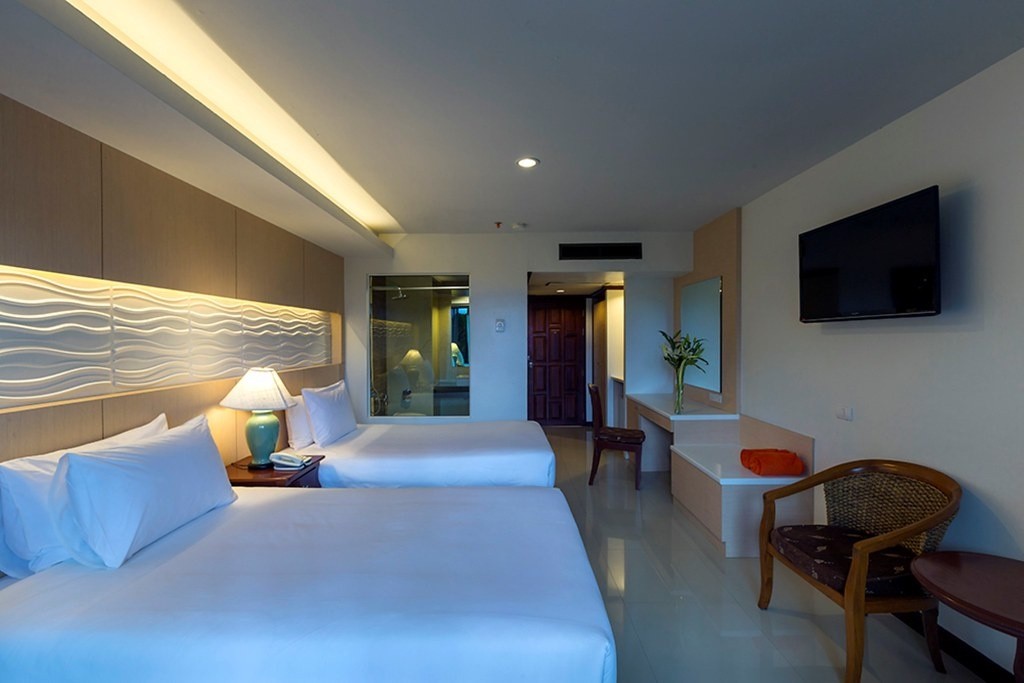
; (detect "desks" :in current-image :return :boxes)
[611,376,624,429]
[626,392,739,472]
[910,550,1024,683]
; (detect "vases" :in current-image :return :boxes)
[674,368,684,415]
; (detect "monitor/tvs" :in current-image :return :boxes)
[797,184,942,324]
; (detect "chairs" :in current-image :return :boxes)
[588,383,645,490]
[757,459,963,683]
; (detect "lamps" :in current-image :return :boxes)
[218,368,298,470]
[399,349,426,388]
[451,343,459,367]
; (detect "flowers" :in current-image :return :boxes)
[658,328,709,415]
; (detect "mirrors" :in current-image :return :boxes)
[451,303,470,368]
[681,276,723,393]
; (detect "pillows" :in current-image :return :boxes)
[46,413,238,571]
[286,394,314,452]
[0,413,168,580]
[301,378,357,448]
[392,360,434,388]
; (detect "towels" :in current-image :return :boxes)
[740,449,806,477]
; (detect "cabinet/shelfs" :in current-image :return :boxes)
[670,444,814,558]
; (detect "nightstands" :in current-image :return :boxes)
[226,455,326,488]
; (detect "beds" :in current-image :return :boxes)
[0,362,619,683]
